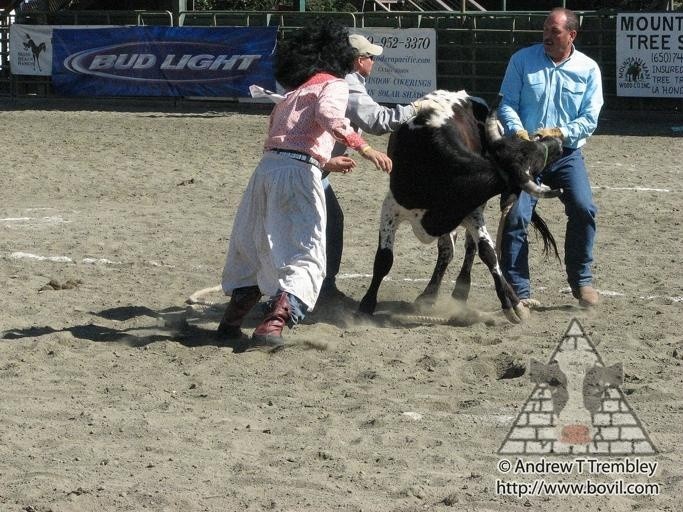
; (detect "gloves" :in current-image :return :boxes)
[533,128,562,142]
[515,129,530,141]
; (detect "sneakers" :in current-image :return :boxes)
[572,285,599,306]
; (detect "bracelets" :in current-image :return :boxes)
[359,146,371,157]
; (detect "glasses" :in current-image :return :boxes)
[360,54,374,61]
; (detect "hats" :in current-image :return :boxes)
[348,34,383,56]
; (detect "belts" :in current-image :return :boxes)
[269,149,322,170]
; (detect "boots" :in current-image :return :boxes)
[319,279,354,306]
[215,287,261,338]
[252,292,292,345]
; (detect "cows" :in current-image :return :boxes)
[352,86,566,329]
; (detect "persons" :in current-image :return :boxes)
[259,35,451,315]
[497,8,606,308]
[215,16,395,348]
